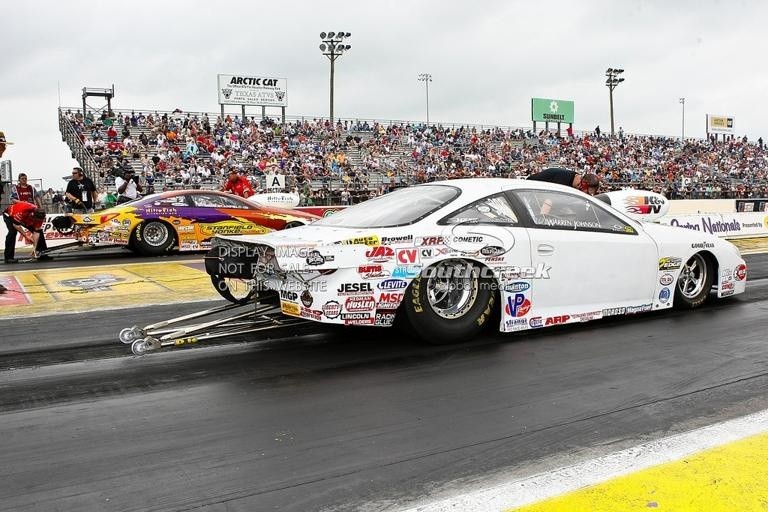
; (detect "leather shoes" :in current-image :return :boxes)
[5,258,18,264]
[38,255,53,260]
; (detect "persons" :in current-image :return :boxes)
[2,202,46,264]
[0,131,14,295]
[9,172,42,210]
[43,186,61,213]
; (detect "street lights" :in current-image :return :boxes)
[604,68,624,138]
[420,72,432,125]
[678,97,685,140]
[319,29,348,123]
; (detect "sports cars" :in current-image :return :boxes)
[69,187,324,264]
[205,177,747,354]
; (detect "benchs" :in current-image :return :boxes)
[59,109,656,195]
